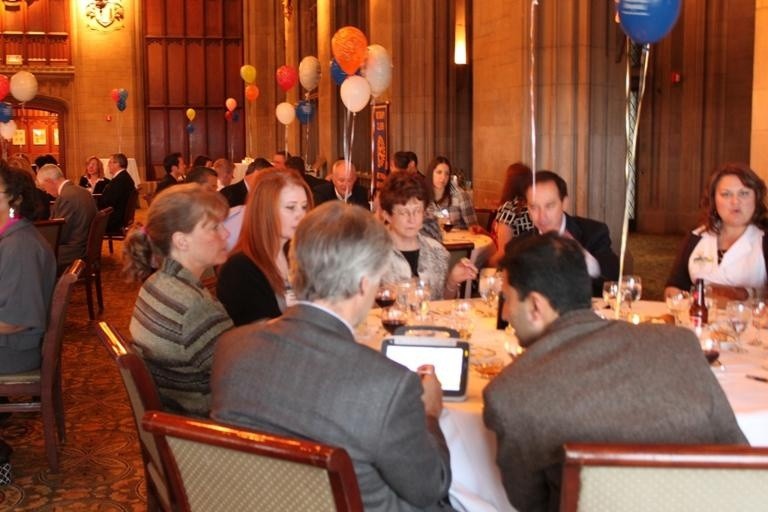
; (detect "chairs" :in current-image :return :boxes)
[98,188,142,256]
[560,443,768,512]
[97,316,172,512]
[1,255,86,473]
[444,244,475,298]
[473,209,492,229]
[143,409,362,512]
[27,214,67,262]
[75,206,115,323]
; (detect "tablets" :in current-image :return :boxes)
[381,338,469,396]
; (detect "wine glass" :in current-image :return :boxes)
[442,221,453,241]
[478,276,499,318]
[666,289,689,330]
[602,276,642,325]
[727,297,765,354]
[452,303,474,343]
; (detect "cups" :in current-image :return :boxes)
[375,279,432,338]
[700,336,720,367]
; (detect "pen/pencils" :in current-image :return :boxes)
[745,375,768,383]
[416,370,431,374]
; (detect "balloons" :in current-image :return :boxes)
[618,0,682,44]
[240,65,256,82]
[225,98,239,121]
[276,26,393,125]
[111,87,128,111]
[245,85,259,100]
[0,70,39,140]
[188,123,194,132]
[186,108,196,121]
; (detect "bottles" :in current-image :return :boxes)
[691,278,708,330]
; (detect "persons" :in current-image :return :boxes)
[663,163,768,301]
[8,154,136,276]
[156,151,620,327]
[211,201,457,512]
[127,183,234,419]
[482,231,752,512]
[1,158,57,478]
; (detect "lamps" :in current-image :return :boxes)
[81,0,125,31]
